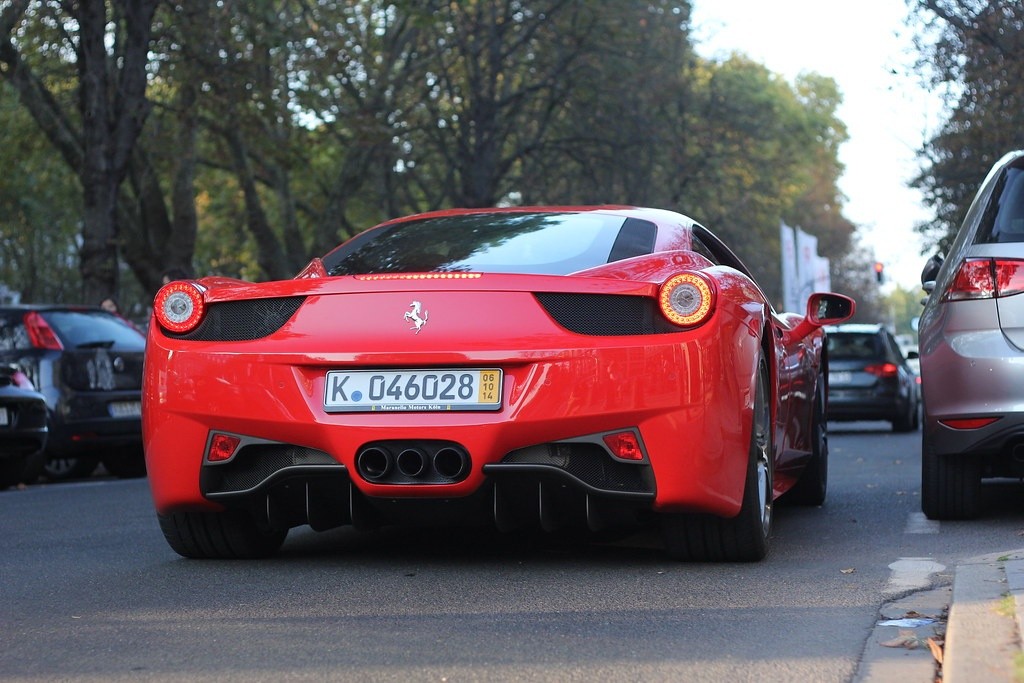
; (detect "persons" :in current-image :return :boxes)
[99,296,119,312]
[161,269,174,286]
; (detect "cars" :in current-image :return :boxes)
[823,323,918,435]
[916,150,1023,521]
[1,362,49,492]
[0,306,144,479]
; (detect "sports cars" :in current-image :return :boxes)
[140,205,855,559]
